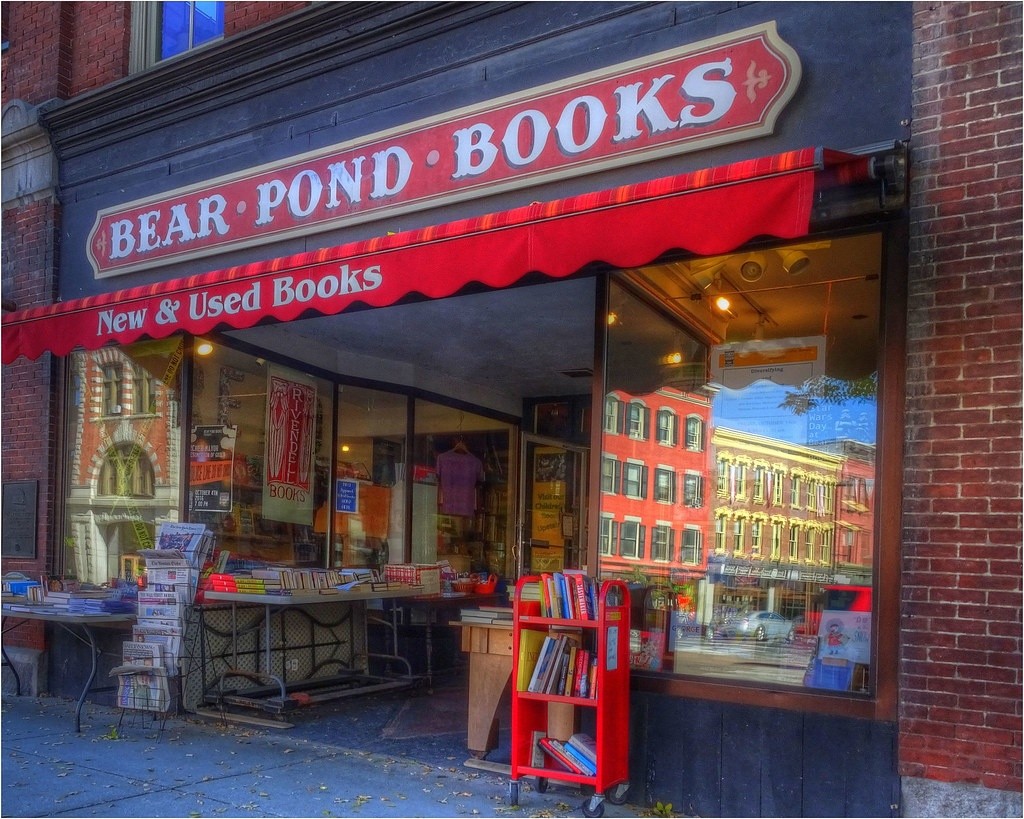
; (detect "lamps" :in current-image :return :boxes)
[739,252,767,283]
[775,249,810,275]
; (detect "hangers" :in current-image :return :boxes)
[453,433,468,453]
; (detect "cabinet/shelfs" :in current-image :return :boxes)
[510,576,630,818]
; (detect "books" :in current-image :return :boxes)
[532,731,597,776]
[517,629,597,700]
[210,563,442,597]
[460,581,540,626]
[437,486,506,576]
[1,576,140,616]
[539,573,618,621]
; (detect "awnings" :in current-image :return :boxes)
[0,147,877,366]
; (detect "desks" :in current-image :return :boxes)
[385,593,507,694]
[1,609,157,734]
[203,589,426,722]
[448,620,513,761]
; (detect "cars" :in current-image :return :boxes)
[725,609,794,641]
